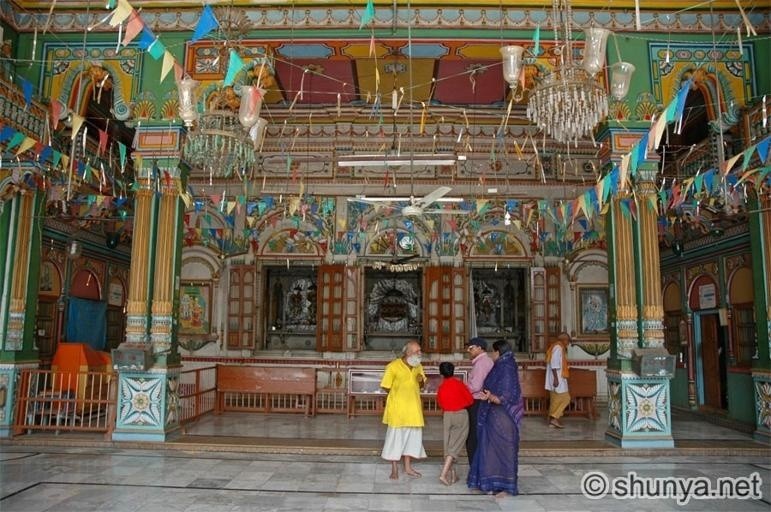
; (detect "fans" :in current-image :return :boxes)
[347,185,472,264]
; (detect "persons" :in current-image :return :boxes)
[440,361,474,486]
[465,336,494,467]
[467,340,526,497]
[379,339,429,479]
[544,331,573,429]
[187,296,203,329]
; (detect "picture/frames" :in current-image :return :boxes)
[576,283,609,337]
[178,279,214,337]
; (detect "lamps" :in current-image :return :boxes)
[175,1,271,186]
[500,0,636,143]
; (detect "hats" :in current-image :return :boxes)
[464,337,487,350]
